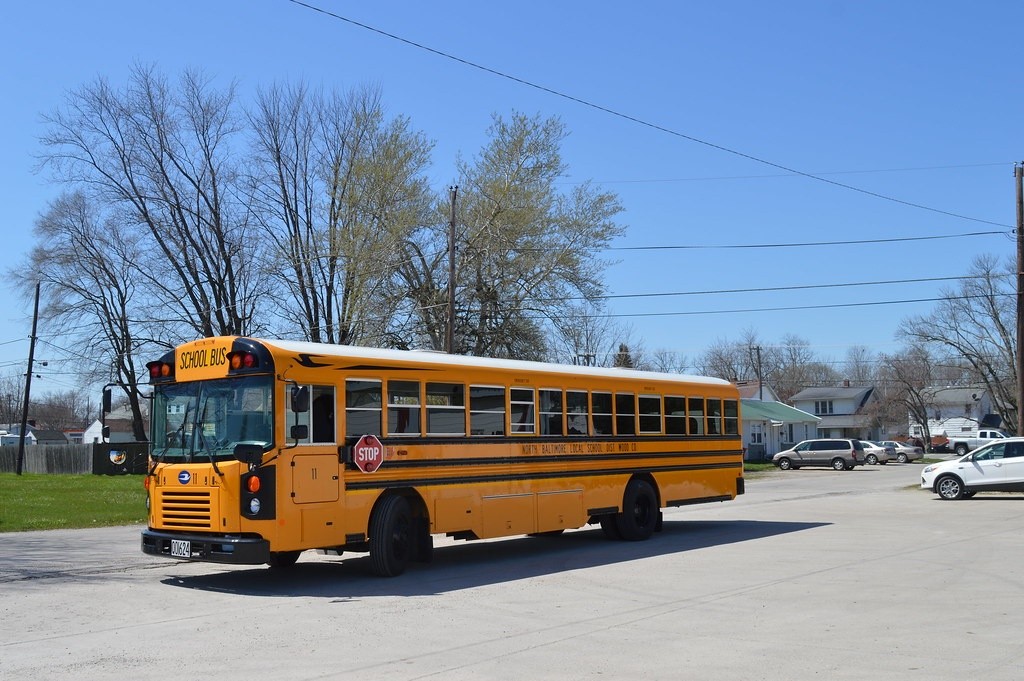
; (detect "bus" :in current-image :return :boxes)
[101,336,746,579]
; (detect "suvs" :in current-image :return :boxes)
[772,438,868,472]
[920,437,1024,500]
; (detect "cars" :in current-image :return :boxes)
[930,441,950,454]
[876,441,924,464]
[858,440,898,465]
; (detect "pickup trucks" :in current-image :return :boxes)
[949,428,1011,456]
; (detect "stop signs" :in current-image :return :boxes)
[353,434,384,475]
